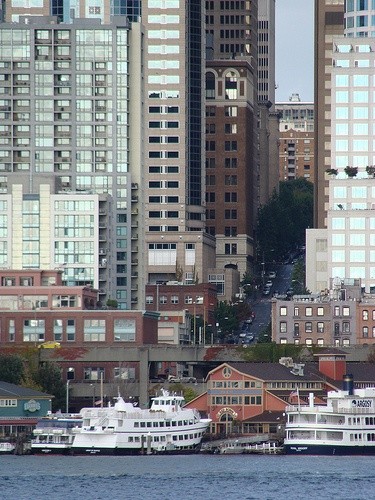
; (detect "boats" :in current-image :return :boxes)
[283,384,375,456]
[0,370,211,456]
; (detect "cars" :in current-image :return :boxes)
[168,374,196,383]
[286,287,294,295]
[239,330,254,340]
[269,272,276,278]
[150,377,165,383]
[272,290,280,297]
[262,281,272,295]
[244,311,256,323]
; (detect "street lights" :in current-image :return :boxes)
[252,263,264,283]
[263,249,274,284]
[239,284,250,299]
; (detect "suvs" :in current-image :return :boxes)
[291,259,298,265]
